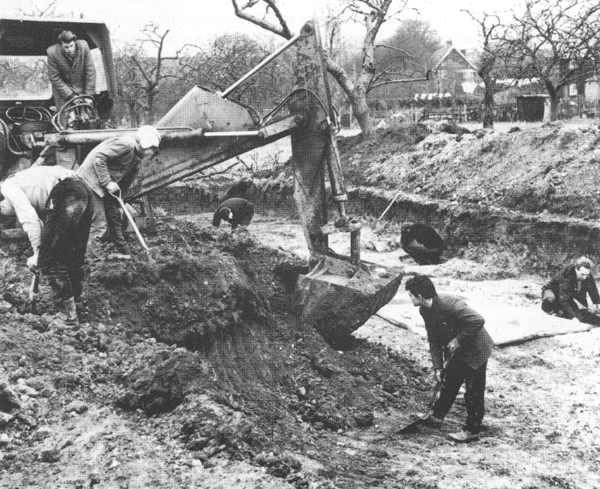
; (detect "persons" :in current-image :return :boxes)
[213,198,254,232]
[0,164,94,330]
[405,276,494,442]
[75,125,161,257]
[46,31,95,130]
[540,256,600,324]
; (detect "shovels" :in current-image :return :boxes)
[396,344,456,434]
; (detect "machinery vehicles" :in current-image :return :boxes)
[0,15,405,341]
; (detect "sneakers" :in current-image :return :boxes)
[448,429,480,443]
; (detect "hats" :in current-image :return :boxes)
[137,124,160,149]
